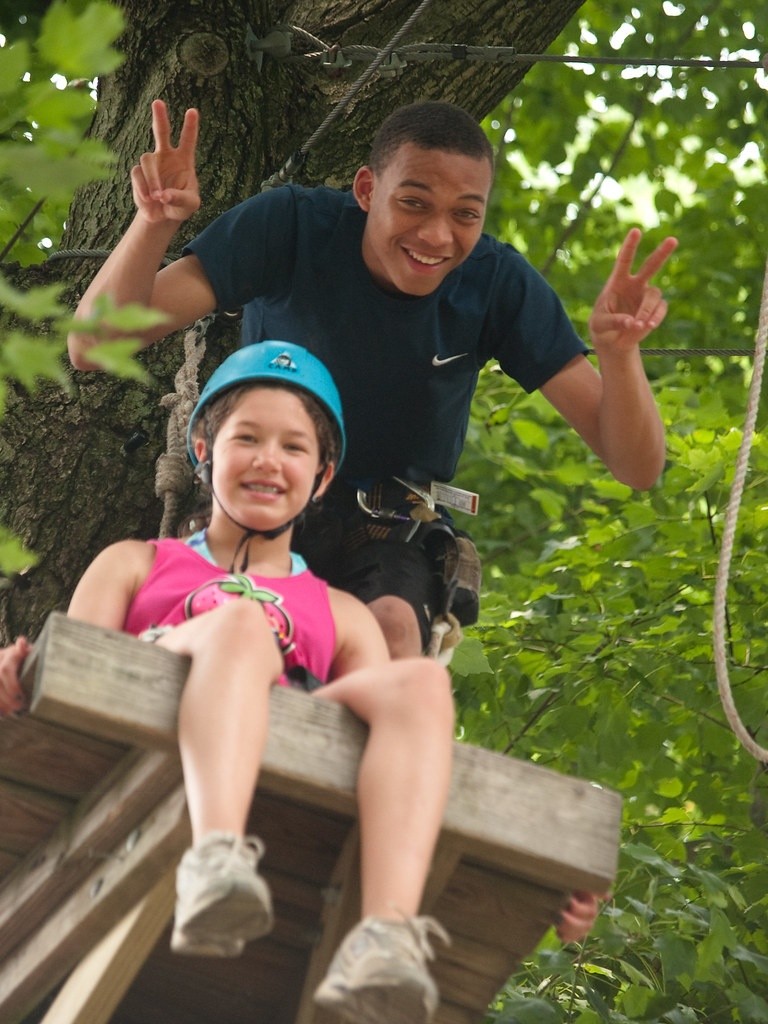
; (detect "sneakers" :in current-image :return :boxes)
[312,905,451,1024]
[170,831,274,957]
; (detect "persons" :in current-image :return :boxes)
[0,342,613,1023]
[67,100,680,658]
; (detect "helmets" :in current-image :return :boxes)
[187,340,345,479]
